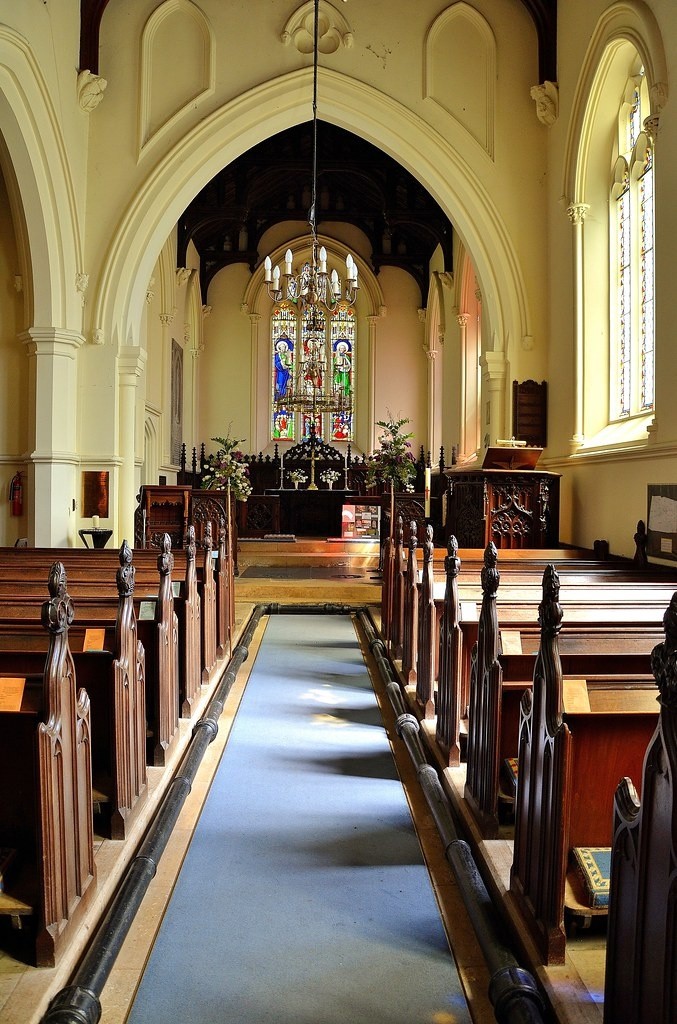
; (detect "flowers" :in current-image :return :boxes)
[285,469,308,483]
[201,437,253,502]
[364,419,415,492]
[319,468,340,483]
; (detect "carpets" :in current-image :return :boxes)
[124,617,475,1024]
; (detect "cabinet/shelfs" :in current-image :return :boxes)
[139,484,191,549]
[444,471,564,549]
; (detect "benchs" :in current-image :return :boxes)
[380,513,677,1024]
[0,516,235,968]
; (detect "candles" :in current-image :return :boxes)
[280,453,282,468]
[90,515,99,528]
[344,451,347,468]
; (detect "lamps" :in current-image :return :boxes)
[263,0,360,416]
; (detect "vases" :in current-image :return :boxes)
[327,481,333,490]
[293,481,298,489]
